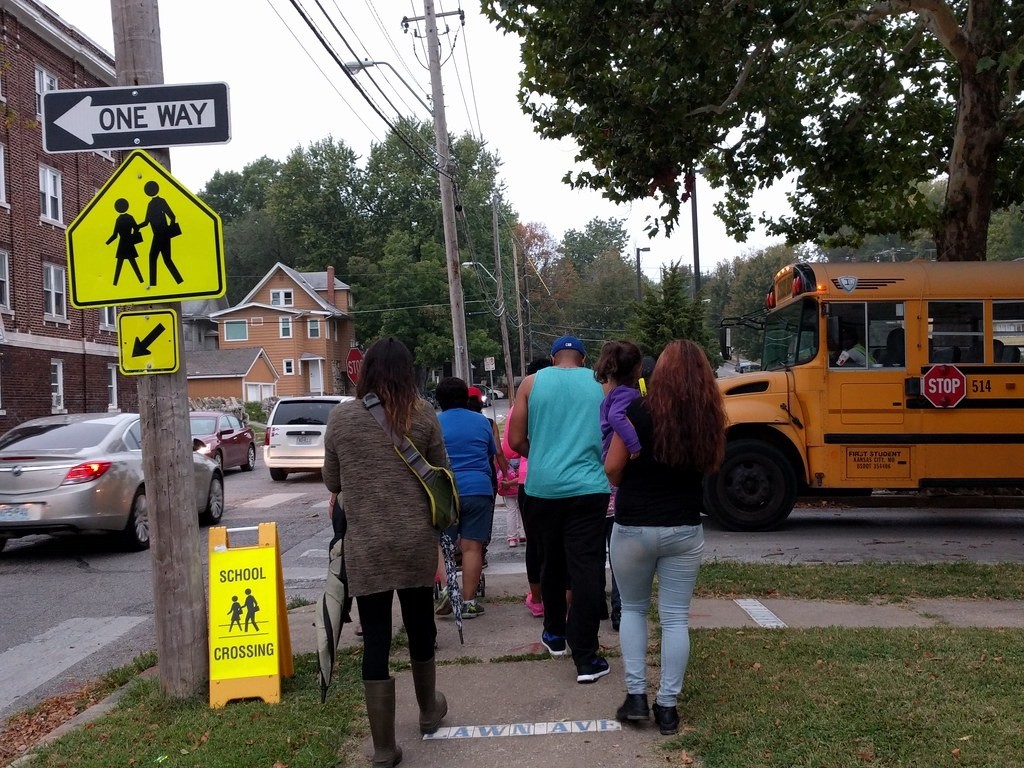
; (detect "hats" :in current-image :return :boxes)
[550,336,586,355]
[468,386,483,402]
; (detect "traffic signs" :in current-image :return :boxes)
[41,80,233,154]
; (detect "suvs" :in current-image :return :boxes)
[263,395,357,481]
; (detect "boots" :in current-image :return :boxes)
[410,653,447,733]
[363,676,403,768]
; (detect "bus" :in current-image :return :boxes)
[701,258,1024,532]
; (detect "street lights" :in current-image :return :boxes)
[343,57,470,387]
[462,261,516,408]
[636,247,651,303]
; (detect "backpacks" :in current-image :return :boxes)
[496,469,519,497]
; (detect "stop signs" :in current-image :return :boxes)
[345,348,365,387]
[923,364,967,409]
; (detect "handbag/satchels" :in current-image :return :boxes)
[422,467,460,531]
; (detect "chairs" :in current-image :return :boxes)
[884,327,1021,364]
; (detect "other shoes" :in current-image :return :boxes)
[481,559,488,568]
[526,592,544,617]
[615,694,650,720]
[611,610,621,632]
[600,604,609,620]
[455,560,462,570]
[509,539,518,546]
[519,538,526,544]
[652,698,680,734]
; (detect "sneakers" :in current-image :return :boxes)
[461,602,485,618]
[577,656,610,682]
[434,586,453,615]
[541,628,567,655]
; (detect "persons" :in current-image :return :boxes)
[594,341,642,460]
[322,337,452,768]
[604,340,725,734]
[501,355,655,633]
[509,338,612,681]
[832,330,877,367]
[435,377,507,619]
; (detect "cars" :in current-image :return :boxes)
[472,383,505,408]
[0,412,225,556]
[189,411,257,473]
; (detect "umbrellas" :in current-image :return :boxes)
[441,532,464,644]
[316,491,351,704]
